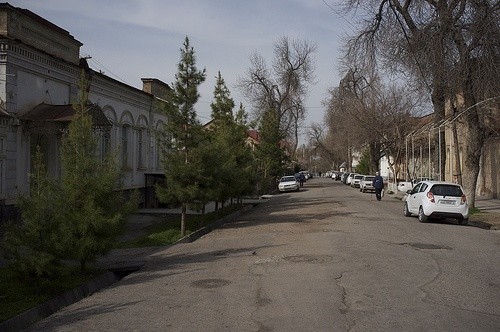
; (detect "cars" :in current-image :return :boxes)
[360,175,377,193]
[326,169,343,180]
[278,175,300,192]
[346,173,359,185]
[295,170,313,182]
[404,181,469,226]
[341,172,349,183]
[350,175,364,188]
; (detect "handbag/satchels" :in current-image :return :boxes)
[381,189,384,198]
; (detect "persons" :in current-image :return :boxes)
[319,170,322,177]
[372,171,384,201]
[299,172,305,188]
